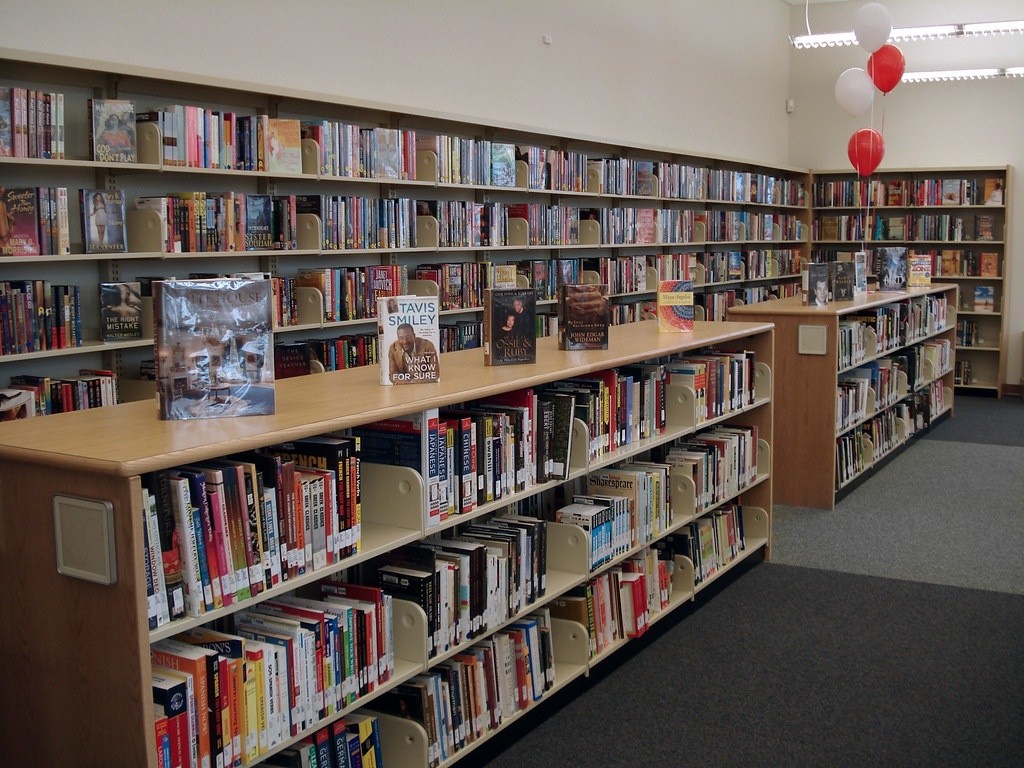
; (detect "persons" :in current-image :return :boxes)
[986,182,1002,206]
[810,279,827,306]
[88,194,114,246]
[836,265,853,298]
[511,298,534,348]
[496,314,520,354]
[388,324,440,384]
[118,111,135,143]
[566,286,608,316]
[98,114,133,150]
[2,404,27,423]
[387,299,400,314]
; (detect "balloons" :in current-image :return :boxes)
[835,68,876,119]
[853,3,893,53]
[848,129,886,179]
[867,44,906,92]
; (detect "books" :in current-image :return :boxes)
[0,84,1007,768]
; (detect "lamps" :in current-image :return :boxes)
[901,67,1024,84]
[789,20,1024,50]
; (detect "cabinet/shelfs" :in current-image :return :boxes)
[1,46,1013,768]
